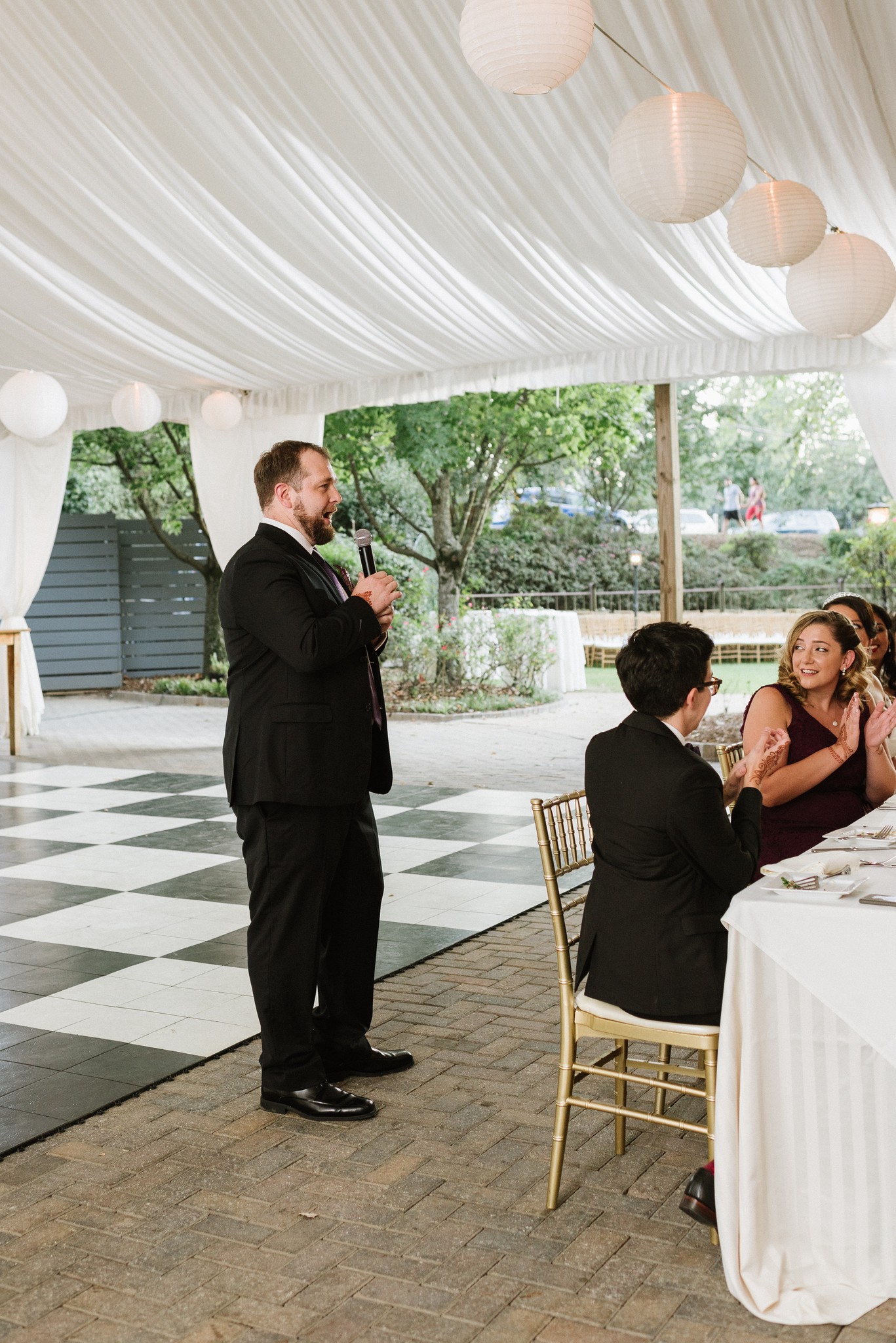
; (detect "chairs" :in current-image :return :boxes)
[531,790,721,1246]
[716,741,745,815]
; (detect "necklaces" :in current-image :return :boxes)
[807,692,838,726]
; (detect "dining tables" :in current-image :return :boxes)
[714,791,896,1326]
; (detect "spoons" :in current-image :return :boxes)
[838,825,893,841]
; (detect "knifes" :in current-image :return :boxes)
[811,846,896,853]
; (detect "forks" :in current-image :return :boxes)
[791,863,853,890]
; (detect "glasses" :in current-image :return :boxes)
[695,677,723,696]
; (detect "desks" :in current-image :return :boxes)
[0,628,31,756]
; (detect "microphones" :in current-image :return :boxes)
[353,528,377,577]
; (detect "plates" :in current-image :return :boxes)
[822,825,896,846]
[760,871,870,899]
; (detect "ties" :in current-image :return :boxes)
[314,551,382,731]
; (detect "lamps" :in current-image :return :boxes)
[0,371,69,438]
[202,390,242,428]
[112,382,161,433]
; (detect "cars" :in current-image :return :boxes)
[492,484,841,535]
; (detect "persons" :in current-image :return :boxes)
[574,621,791,1237]
[868,600,895,703]
[216,439,420,1124]
[735,608,896,885]
[743,476,765,531]
[822,591,896,767]
[713,476,747,533]
